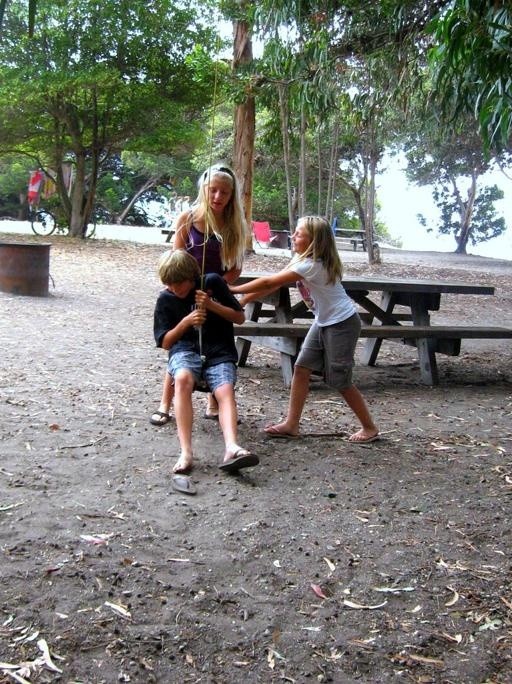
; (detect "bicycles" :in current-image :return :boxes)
[31,207,96,238]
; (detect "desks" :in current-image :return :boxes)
[334,227,367,252]
[231,273,496,392]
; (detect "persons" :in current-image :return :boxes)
[150,161,260,473]
[228,215,379,443]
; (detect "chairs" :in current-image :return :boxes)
[250,220,279,250]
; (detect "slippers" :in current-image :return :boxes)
[218,448,260,471]
[348,429,381,444]
[173,453,195,474]
[204,409,242,424]
[149,406,170,424]
[262,423,300,439]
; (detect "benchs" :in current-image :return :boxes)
[233,308,510,342]
[349,237,364,245]
